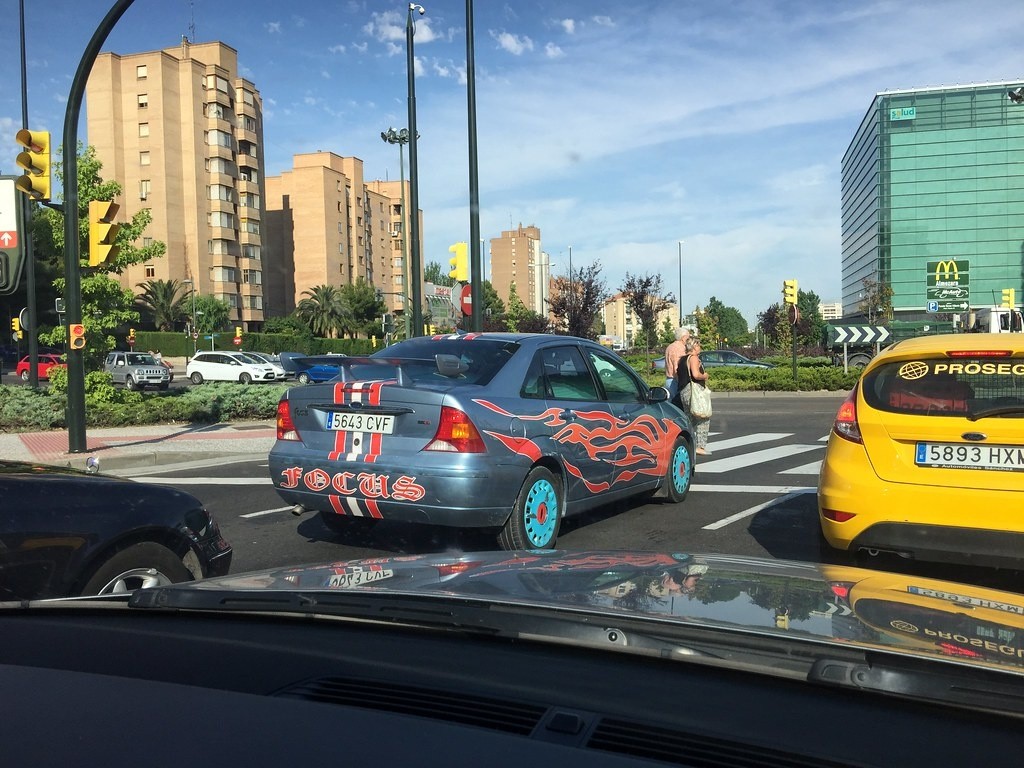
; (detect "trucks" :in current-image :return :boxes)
[818,304,1023,371]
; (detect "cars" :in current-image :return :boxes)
[652,349,777,370]
[816,333,1024,577]
[817,563,1023,671]
[287,552,687,598]
[225,351,295,382]
[17,353,70,382]
[612,344,628,353]
[0,459,234,598]
[268,330,697,552]
[295,351,353,384]
[186,350,277,385]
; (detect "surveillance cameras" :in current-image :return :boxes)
[419,8,425,15]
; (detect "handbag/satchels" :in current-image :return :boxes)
[690,381,712,418]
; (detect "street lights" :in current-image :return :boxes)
[679,241,686,329]
[382,126,421,337]
[568,245,573,321]
[406,3,426,333]
[480,238,486,321]
[527,263,556,322]
[183,279,204,352]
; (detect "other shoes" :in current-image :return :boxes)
[696,449,712,455]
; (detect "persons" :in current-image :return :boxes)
[676,338,712,455]
[664,328,690,403]
[156,349,162,362]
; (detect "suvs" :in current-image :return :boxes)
[104,351,171,390]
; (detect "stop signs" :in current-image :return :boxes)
[788,304,797,325]
[234,336,242,345]
[129,337,135,346]
[460,285,472,316]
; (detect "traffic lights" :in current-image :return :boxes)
[784,279,797,304]
[236,327,242,336]
[131,329,137,337]
[1001,289,1015,309]
[89,200,121,268]
[448,242,469,281]
[69,326,87,349]
[14,129,50,203]
